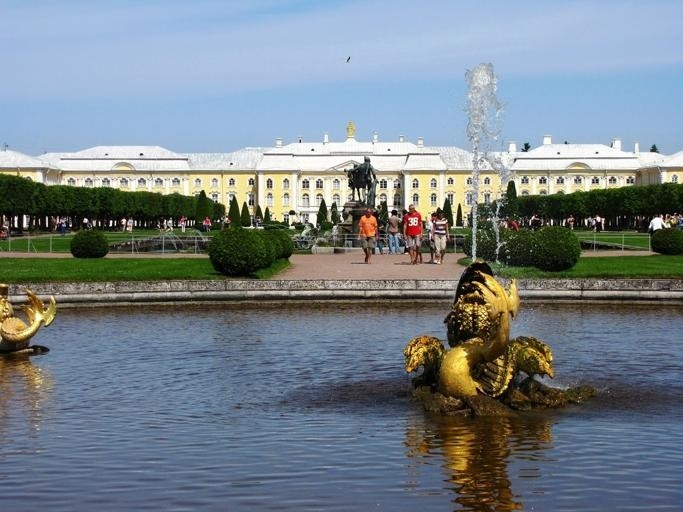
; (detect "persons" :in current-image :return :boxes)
[344,163,365,203]
[356,157,377,203]
[499,211,683,236]
[0,214,233,240]
[351,198,450,264]
